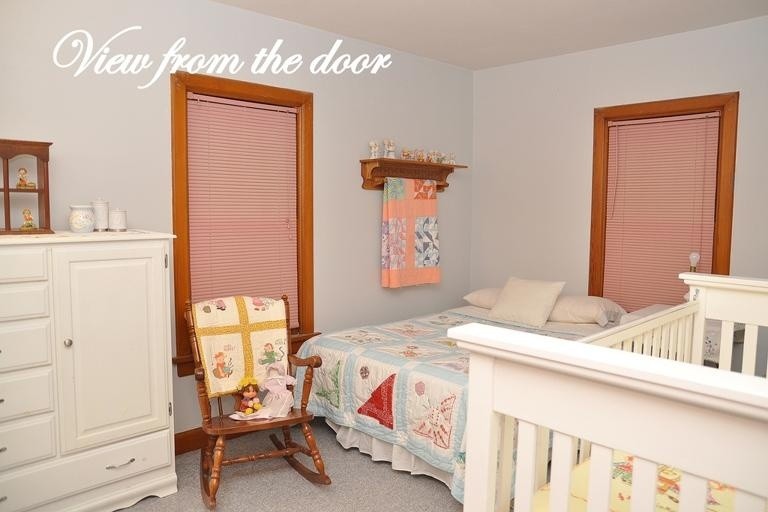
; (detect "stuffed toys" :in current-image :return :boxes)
[228,362,297,421]
[22,209,35,228]
[16,167,28,187]
[236,376,263,413]
[368,139,456,164]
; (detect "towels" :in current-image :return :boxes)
[380,177,441,289]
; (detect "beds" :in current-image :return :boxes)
[293,305,622,506]
[447,270,768,512]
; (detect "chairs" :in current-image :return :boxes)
[184,295,331,512]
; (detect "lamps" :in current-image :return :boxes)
[683,252,701,302]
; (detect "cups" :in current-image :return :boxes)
[94,198,127,232]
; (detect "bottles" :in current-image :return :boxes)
[70,205,94,233]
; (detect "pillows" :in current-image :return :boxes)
[462,277,627,328]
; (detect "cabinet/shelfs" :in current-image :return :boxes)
[360,158,456,193]
[0,138,57,234]
[0,230,178,511]
